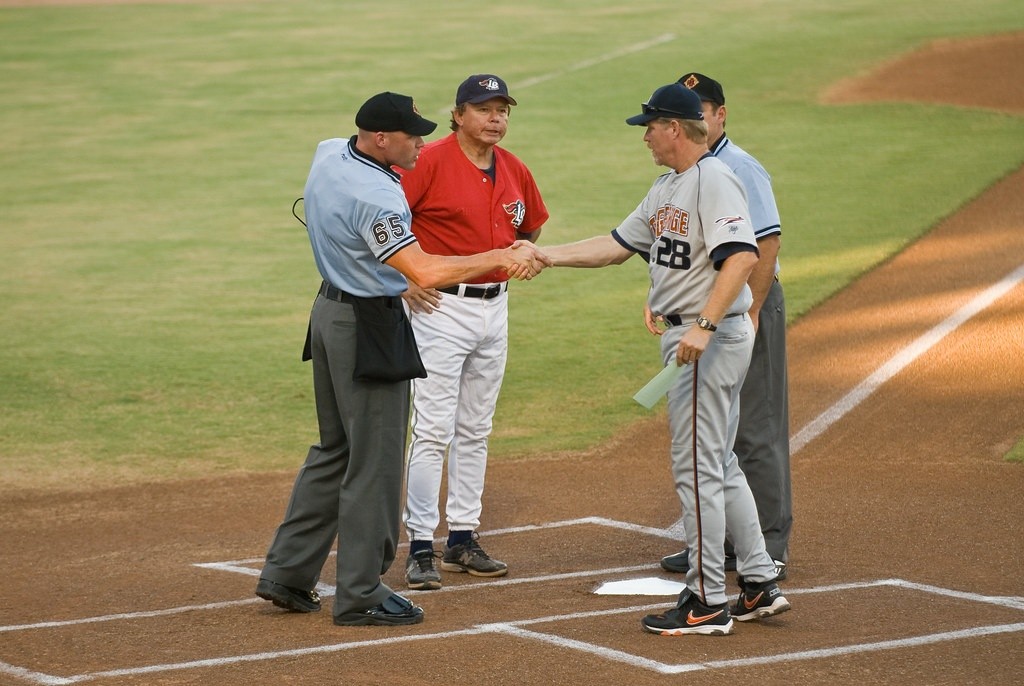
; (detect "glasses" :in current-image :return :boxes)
[642,103,683,115]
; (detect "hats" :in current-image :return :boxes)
[626,82,704,127]
[456,74,518,106]
[676,72,724,102]
[354,91,437,136]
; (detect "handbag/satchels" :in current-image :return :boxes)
[350,299,428,383]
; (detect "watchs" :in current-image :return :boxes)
[696,316,717,332]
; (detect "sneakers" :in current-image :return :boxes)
[255,578,322,612]
[441,532,509,577]
[660,549,736,572]
[727,574,791,621]
[404,547,445,589]
[737,558,787,581]
[333,593,423,625]
[643,587,734,635]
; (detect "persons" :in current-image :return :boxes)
[392,73,550,589]
[255,91,555,626]
[507,81,792,636]
[643,72,792,582]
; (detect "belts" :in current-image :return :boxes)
[434,282,509,299]
[319,282,351,303]
[668,312,742,324]
